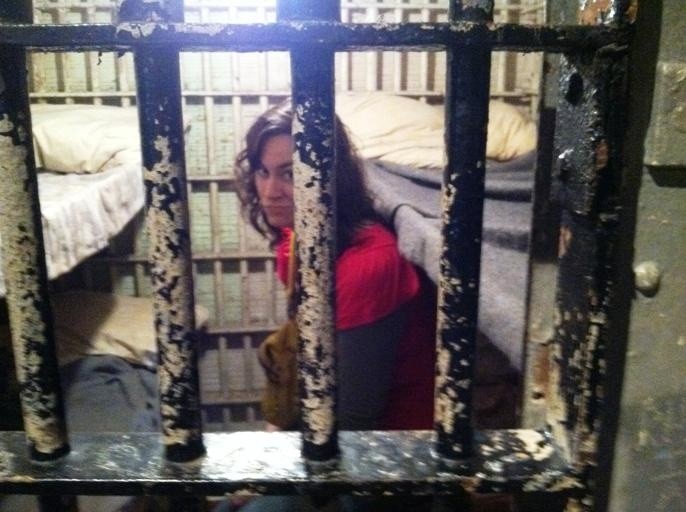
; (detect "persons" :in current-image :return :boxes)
[231,95,438,432]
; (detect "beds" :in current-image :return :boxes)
[352,146,539,431]
[1,176,165,512]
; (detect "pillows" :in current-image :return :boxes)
[26,102,190,174]
[46,289,211,371]
[333,89,539,169]
